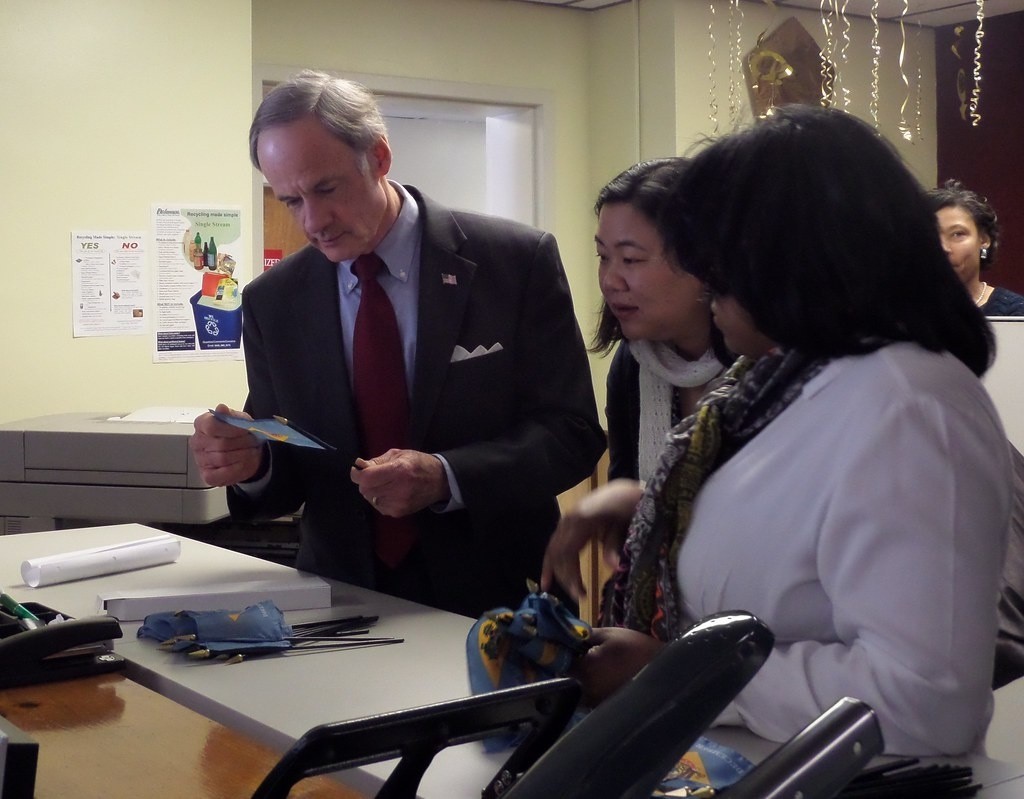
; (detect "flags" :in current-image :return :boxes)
[136,600,293,662]
[209,409,327,451]
[462,588,759,792]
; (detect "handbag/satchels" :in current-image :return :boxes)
[991,433,1023,691]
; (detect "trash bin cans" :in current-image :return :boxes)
[190,288,243,350]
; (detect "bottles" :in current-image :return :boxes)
[185,229,218,270]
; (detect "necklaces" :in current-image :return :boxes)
[974,281,987,306]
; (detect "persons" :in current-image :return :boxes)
[538,100,1010,761]
[372,496,377,506]
[188,69,605,621]
[917,179,1024,322]
[580,155,742,573]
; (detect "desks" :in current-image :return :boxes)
[0,615,373,798]
[0,522,1023,799]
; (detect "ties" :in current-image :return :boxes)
[350,253,420,566]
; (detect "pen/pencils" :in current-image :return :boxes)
[0,589,48,627]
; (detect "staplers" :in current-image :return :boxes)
[0,614,125,689]
